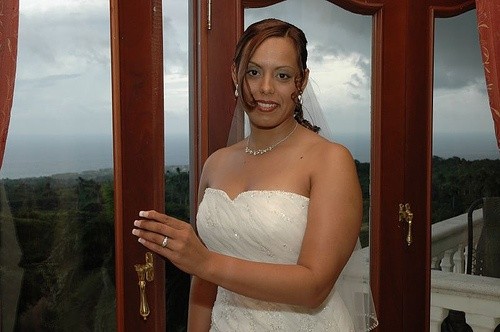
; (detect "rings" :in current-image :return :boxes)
[162,236,168,247]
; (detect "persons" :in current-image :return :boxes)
[131,18,363,332]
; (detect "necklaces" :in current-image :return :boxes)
[245,119,298,155]
